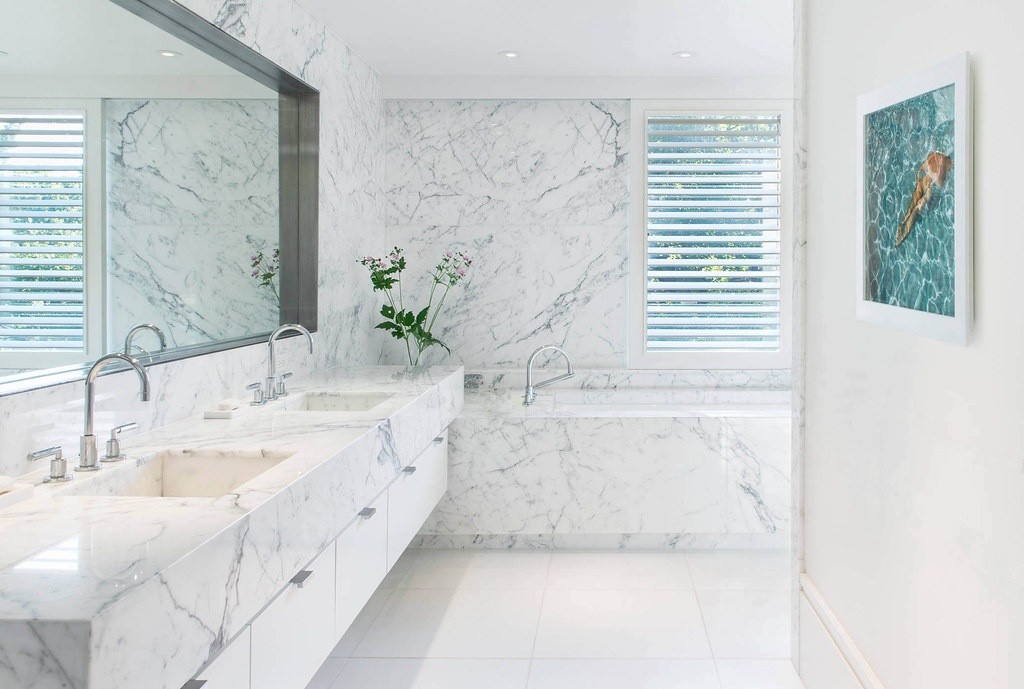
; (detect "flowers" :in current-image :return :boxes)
[355,245,473,366]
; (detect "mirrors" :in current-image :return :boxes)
[0,0,299,387]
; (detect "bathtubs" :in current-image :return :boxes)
[407,390,793,551]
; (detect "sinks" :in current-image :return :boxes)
[60,447,297,499]
[260,391,397,413]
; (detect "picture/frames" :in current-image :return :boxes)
[852,46,977,350]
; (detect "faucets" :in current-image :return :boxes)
[117,345,146,354]
[265,323,314,401]
[522,344,575,407]
[79,352,151,466]
[125,323,167,355]
